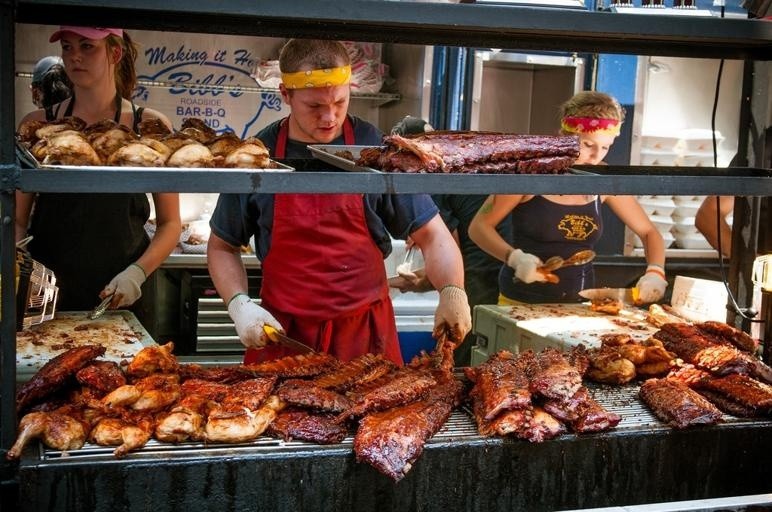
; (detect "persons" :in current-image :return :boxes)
[27,55,75,109]
[12,24,181,323]
[467,90,669,306]
[695,124,772,331]
[207,36,472,367]
[390,115,513,368]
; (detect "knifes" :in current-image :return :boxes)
[261,324,320,358]
[577,286,641,305]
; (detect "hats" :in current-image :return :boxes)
[50,26,124,42]
[31,55,69,84]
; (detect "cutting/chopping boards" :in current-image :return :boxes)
[515,314,659,349]
[14,315,148,375]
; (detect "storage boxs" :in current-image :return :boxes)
[377,238,444,363]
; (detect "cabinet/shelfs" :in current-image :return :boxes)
[0,0,772,194]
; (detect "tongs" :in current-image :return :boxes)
[87,295,115,321]
[536,251,598,276]
[401,246,417,272]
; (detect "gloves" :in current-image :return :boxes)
[99,263,147,309]
[635,263,669,306]
[433,286,472,349]
[508,249,559,285]
[228,293,286,350]
